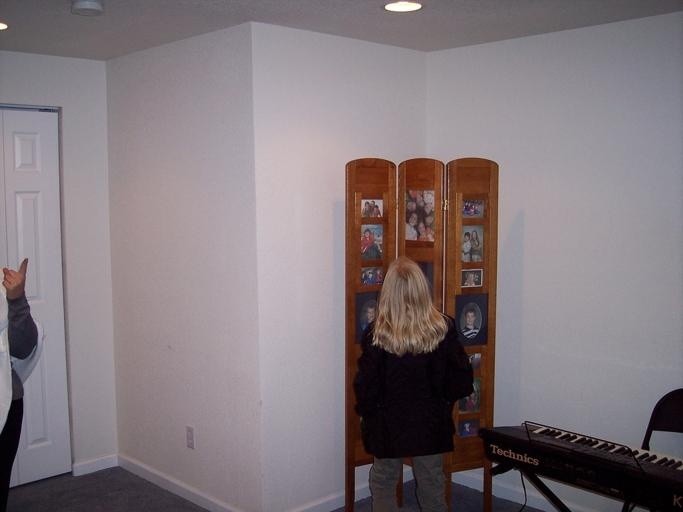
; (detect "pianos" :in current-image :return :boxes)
[479,421,683,511]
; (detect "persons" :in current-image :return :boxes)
[360,268,367,284]
[366,271,376,285]
[464,393,474,413]
[357,295,378,338]
[0,255,45,512]
[463,272,475,287]
[357,187,485,262]
[460,306,478,340]
[468,424,478,435]
[461,420,472,435]
[350,255,476,512]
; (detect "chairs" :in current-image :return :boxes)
[620,387,682,511]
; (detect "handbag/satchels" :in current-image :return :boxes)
[439,336,474,400]
